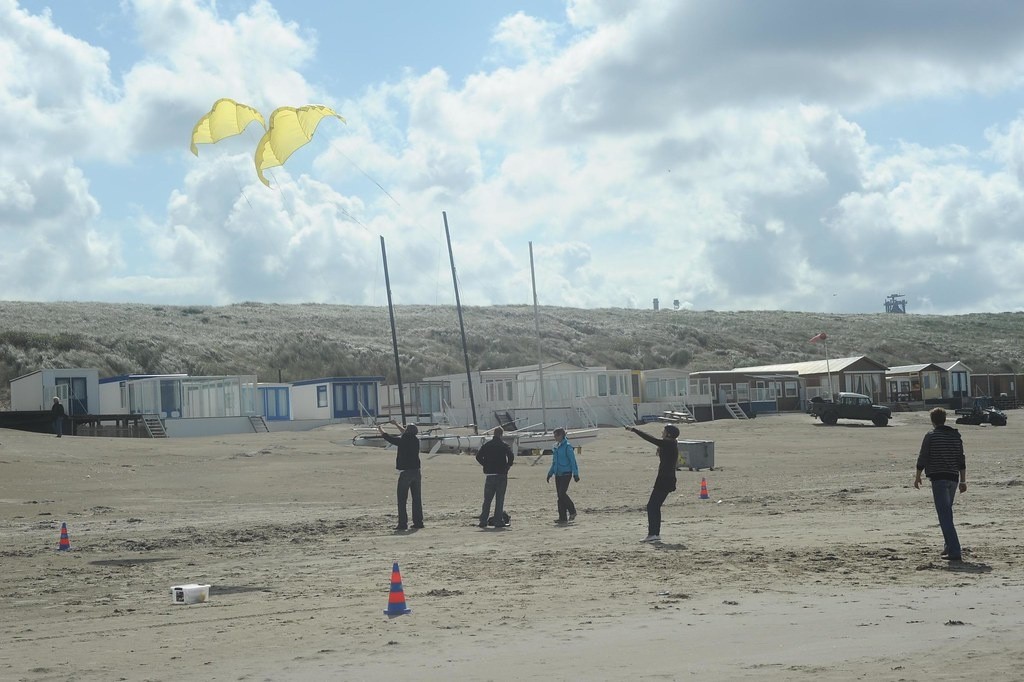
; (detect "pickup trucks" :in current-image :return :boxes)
[807,391,892,427]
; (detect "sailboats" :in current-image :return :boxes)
[498,241,598,451]
[417,211,517,454]
[355,234,432,447]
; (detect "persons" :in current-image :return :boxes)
[914,408,967,560]
[376,419,424,529]
[546,428,580,522]
[624,425,680,542]
[51,397,64,438]
[476,426,514,527]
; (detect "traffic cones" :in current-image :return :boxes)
[56,522,72,551]
[698,477,710,499]
[383,563,411,614]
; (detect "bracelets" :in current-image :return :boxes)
[959,482,966,484]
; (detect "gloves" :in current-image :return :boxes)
[625,425,633,431]
[547,474,551,482]
[573,476,580,481]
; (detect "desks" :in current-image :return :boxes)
[660,411,693,424]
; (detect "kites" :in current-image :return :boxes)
[190,98,266,157]
[255,104,347,190]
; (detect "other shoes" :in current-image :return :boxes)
[639,533,661,542]
[495,522,510,527]
[569,511,577,521]
[940,550,948,556]
[554,517,568,523]
[941,554,951,559]
[478,522,486,527]
[392,525,407,529]
[411,524,425,529]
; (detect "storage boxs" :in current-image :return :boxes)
[170,584,211,604]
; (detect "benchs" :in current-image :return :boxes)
[658,416,679,421]
[673,416,694,422]
